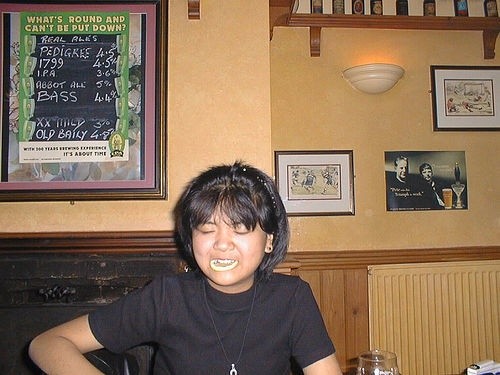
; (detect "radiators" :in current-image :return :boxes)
[367,259,500,375]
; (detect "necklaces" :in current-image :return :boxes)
[201,278,257,375]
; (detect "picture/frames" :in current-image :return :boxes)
[0,0,169,202]
[274,150,356,216]
[430,65,500,132]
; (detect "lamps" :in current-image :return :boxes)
[342,63,405,95]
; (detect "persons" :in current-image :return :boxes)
[28,161,344,375]
[386,156,447,209]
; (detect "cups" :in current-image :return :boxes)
[356,349,399,375]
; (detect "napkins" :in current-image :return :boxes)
[467,360,500,375]
